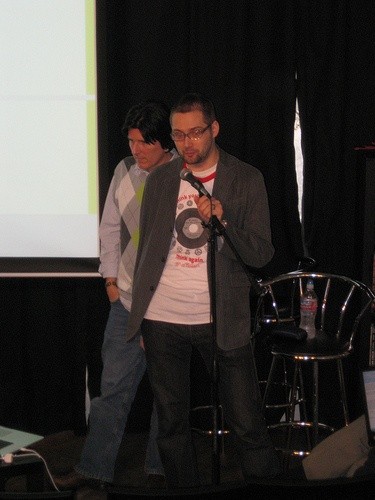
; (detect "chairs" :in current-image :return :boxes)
[252,254,318,453]
[259,271,375,474]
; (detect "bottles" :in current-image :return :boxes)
[300,280,318,338]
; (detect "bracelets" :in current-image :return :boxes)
[105,281,116,287]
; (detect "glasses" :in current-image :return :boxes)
[169,125,209,141]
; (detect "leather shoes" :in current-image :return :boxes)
[140,474,163,488]
[49,471,110,491]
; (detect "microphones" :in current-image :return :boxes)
[180,167,211,199]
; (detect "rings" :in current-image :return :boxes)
[212,204,216,210]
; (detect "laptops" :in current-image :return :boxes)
[0,424,45,459]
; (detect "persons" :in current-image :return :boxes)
[48,101,182,499]
[124,95,286,492]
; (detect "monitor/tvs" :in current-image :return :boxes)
[359,366,375,439]
